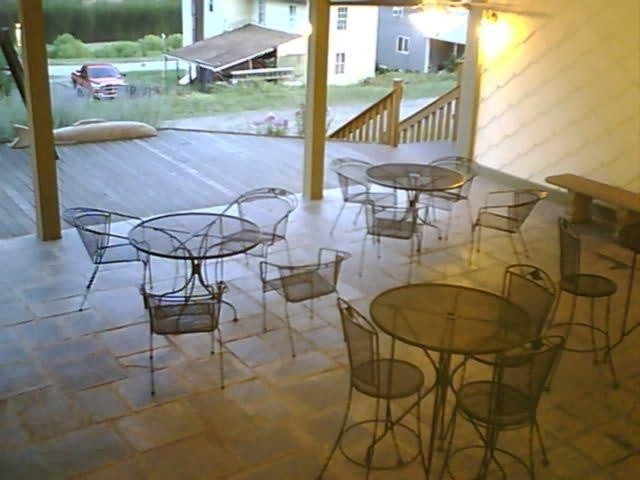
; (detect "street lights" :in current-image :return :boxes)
[159,30,167,88]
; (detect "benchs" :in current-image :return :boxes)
[546,173,637,239]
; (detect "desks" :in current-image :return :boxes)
[127,213,264,323]
[364,284,552,469]
[367,162,466,242]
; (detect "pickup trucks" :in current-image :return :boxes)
[71,61,136,102]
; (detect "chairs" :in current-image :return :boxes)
[138,280,231,397]
[357,198,422,284]
[259,247,353,360]
[436,334,567,479]
[461,261,556,386]
[417,155,483,241]
[214,186,299,282]
[545,214,624,392]
[313,296,427,480]
[60,205,152,312]
[327,155,395,243]
[467,188,549,276]
